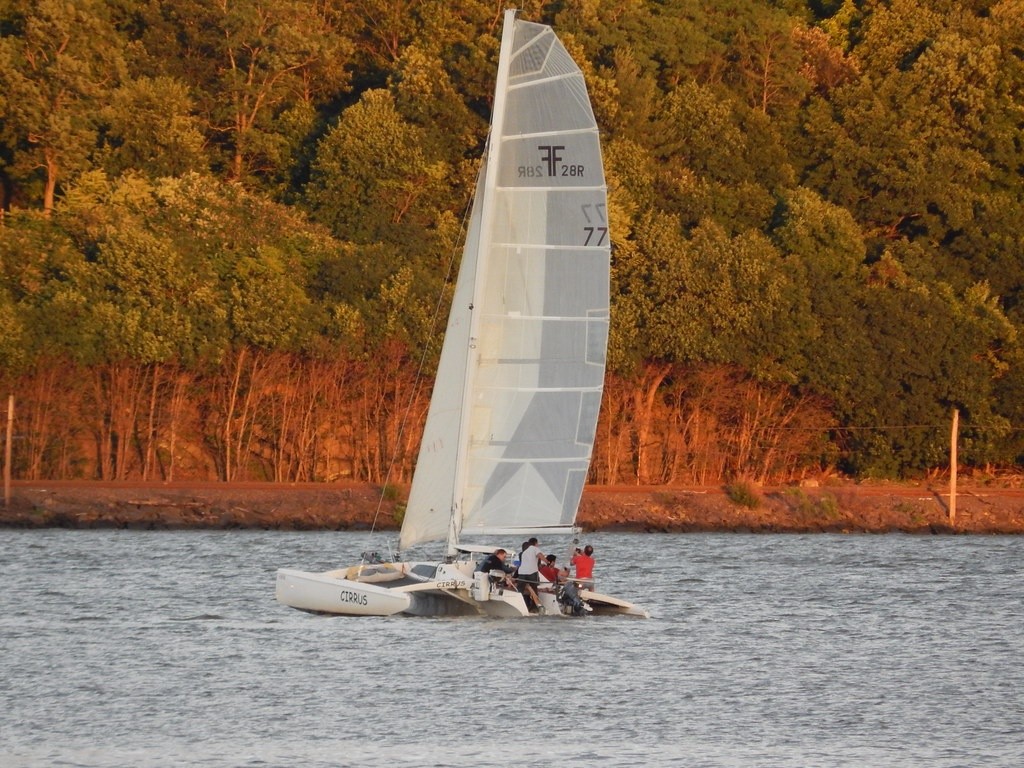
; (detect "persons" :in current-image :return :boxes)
[570,545,595,591]
[472,537,571,612]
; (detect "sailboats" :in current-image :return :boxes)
[276,8,652,621]
